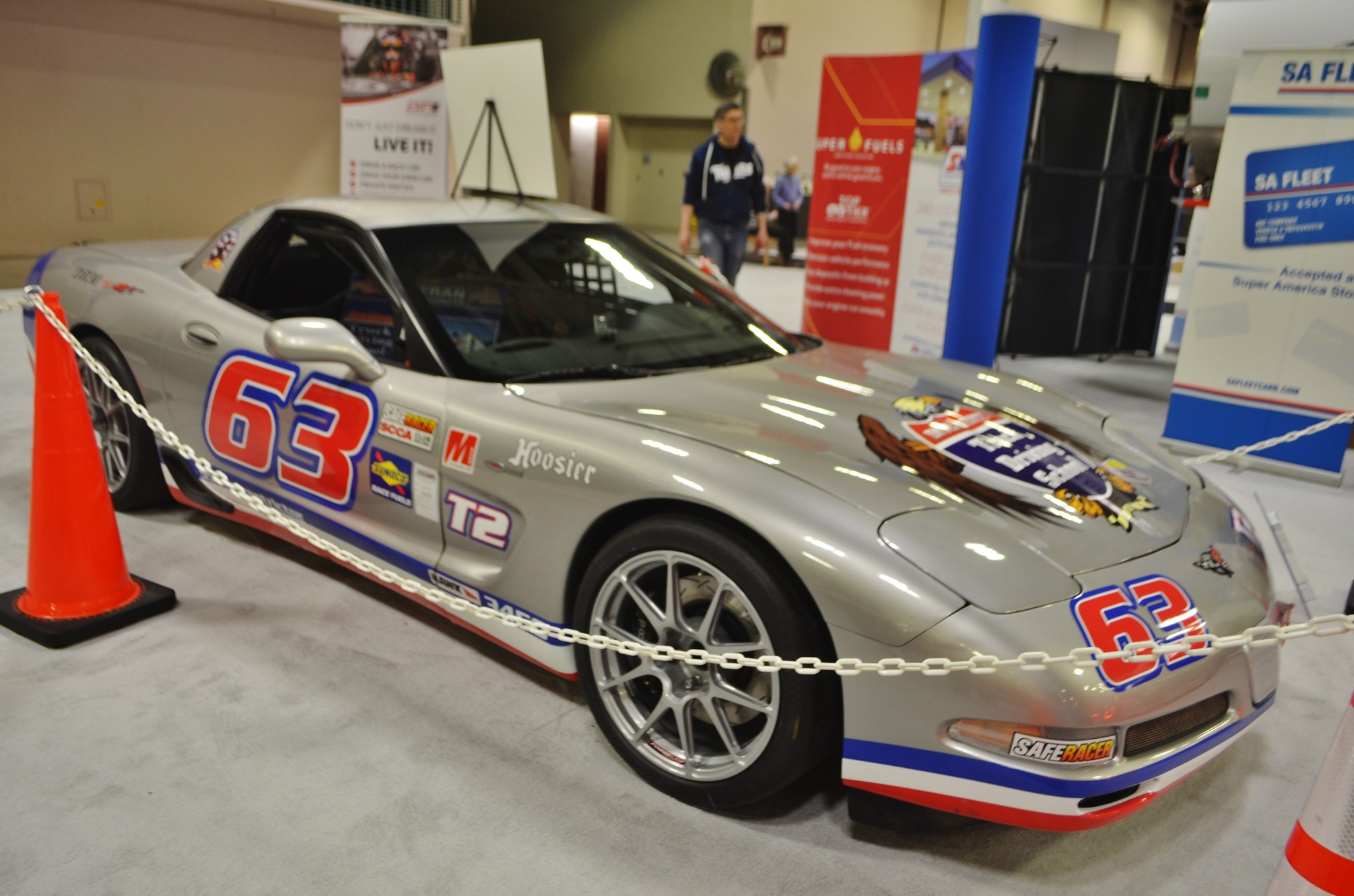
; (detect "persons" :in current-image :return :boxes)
[772,156,804,268]
[677,102,771,287]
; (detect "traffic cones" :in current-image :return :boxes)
[0,291,178,650]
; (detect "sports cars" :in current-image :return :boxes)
[20,195,1285,838]
